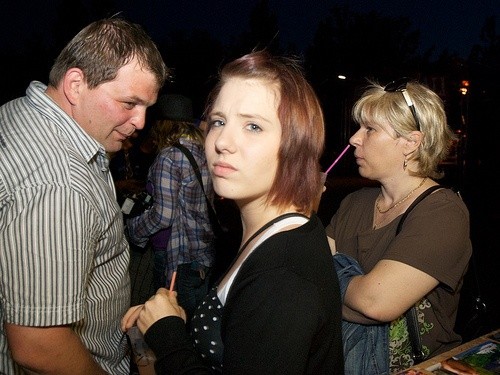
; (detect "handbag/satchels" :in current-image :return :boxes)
[167,141,243,271]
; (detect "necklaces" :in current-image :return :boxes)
[373,176,426,229]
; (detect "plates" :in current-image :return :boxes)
[441,341,500,375]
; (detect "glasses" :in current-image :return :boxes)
[381,76,423,130]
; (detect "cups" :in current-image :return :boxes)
[127,323,158,375]
[318,172,328,205]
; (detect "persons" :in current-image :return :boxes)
[0,10,171,375]
[119,48,346,375]
[108,94,219,335]
[321,73,474,375]
